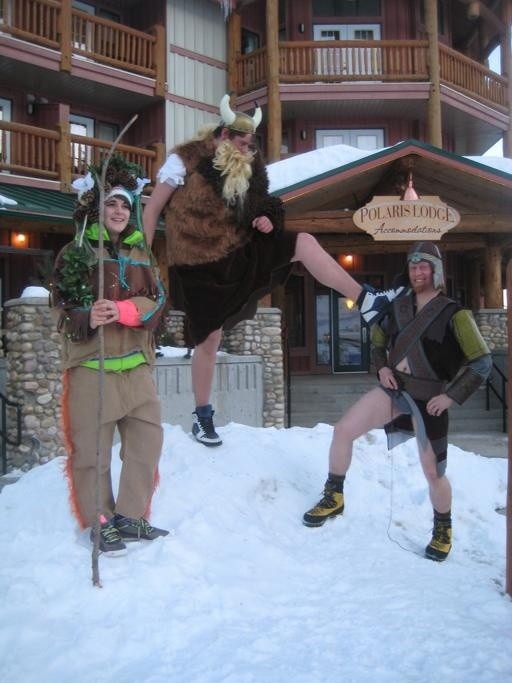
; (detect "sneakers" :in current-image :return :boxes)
[116,514,169,541]
[192,404,222,448]
[358,283,407,326]
[91,524,127,556]
[303,490,344,527]
[425,526,451,560]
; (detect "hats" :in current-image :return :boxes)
[102,183,134,209]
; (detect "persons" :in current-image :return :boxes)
[52,153,170,555]
[302,237,496,562]
[142,112,408,448]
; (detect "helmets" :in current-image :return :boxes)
[221,91,263,134]
[393,241,445,289]
